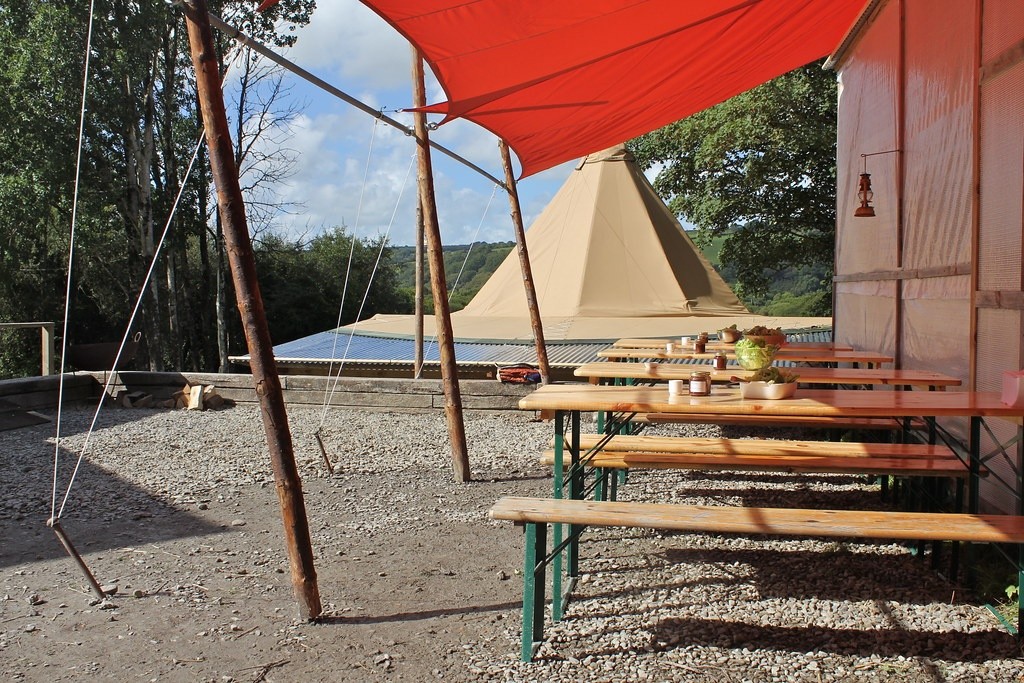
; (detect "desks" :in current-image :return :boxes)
[518,338,1024,623]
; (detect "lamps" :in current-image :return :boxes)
[855,148,901,217]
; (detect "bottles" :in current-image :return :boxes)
[697,332,708,343]
[713,353,726,371]
[694,339,705,354]
[688,371,712,396]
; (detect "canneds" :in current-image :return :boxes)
[713,352,727,370]
[693,332,708,354]
[689,371,711,396]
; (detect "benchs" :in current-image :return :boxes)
[488,414,1024,664]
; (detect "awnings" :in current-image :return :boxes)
[46,0,875,530]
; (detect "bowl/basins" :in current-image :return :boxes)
[717,331,742,343]
[644,362,658,368]
[734,348,778,372]
[743,334,786,349]
[739,380,797,400]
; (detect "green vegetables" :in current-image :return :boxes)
[717,324,800,384]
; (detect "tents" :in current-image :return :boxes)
[329,136,836,346]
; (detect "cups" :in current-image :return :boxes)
[669,380,683,395]
[682,337,691,346]
[667,343,675,352]
